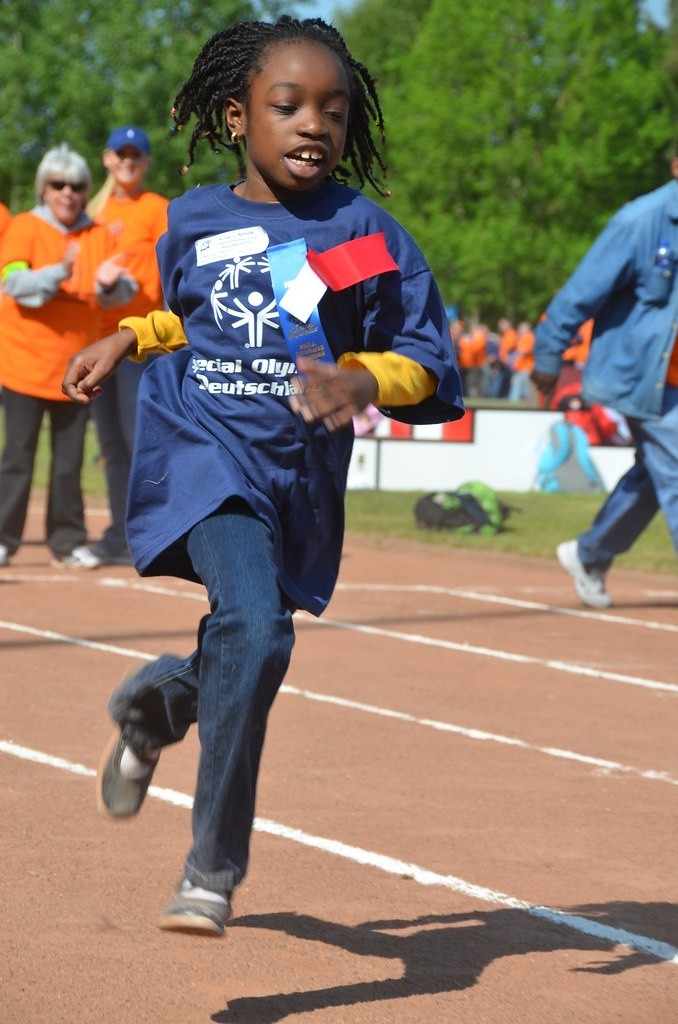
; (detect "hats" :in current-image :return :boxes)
[107,127,150,155]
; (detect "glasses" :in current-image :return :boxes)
[47,181,88,193]
[115,151,144,160]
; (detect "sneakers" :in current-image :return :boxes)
[557,540,613,608]
[0,544,10,566]
[96,726,161,819]
[50,547,101,570]
[159,876,231,935]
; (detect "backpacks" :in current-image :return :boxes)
[535,420,604,492]
[415,482,522,537]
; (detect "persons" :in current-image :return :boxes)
[0,129,593,575]
[62,16,465,935]
[529,153,678,609]
